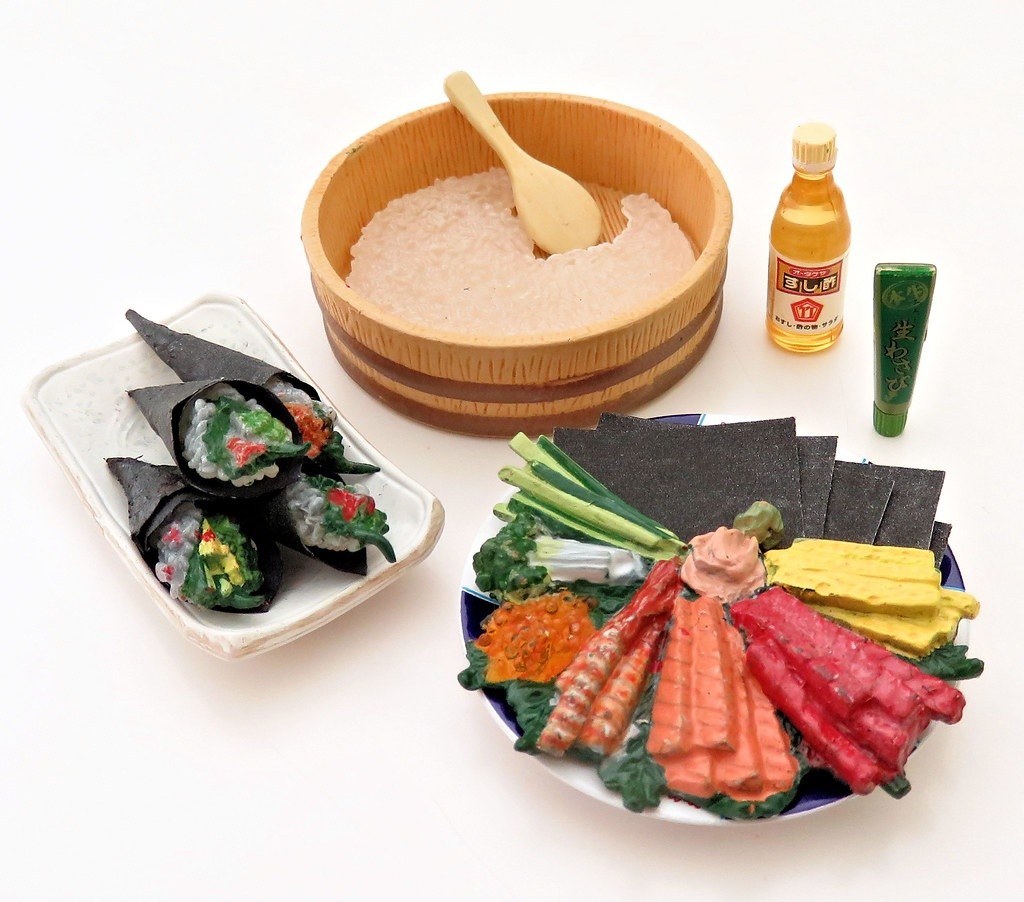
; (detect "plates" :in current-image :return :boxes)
[461,414,965,826]
[31,299,446,663]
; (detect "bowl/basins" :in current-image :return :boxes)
[301,94,733,437]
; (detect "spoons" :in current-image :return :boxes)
[446,71,603,256]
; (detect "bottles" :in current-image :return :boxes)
[766,123,852,355]
[870,262,939,440]
